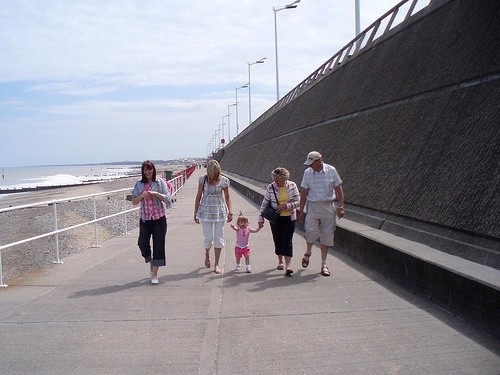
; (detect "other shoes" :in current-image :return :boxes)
[236,264,241,271]
[277,262,283,270]
[149,271,152,279]
[246,265,252,273]
[151,278,159,284]
[214,266,222,273]
[205,257,211,268]
[286,269,293,276]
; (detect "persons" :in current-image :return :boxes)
[228,212,263,272]
[193,160,233,273]
[131,161,171,284]
[256,166,302,274]
[297,150,344,275]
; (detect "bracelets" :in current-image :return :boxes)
[154,192,158,196]
[338,207,344,210]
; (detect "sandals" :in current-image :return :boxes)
[302,253,311,268]
[321,266,330,276]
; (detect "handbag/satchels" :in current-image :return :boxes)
[261,183,280,223]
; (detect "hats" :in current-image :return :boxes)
[304,151,322,165]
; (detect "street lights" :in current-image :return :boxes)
[215,104,238,154]
[272,0,302,102]
[248,57,267,125]
[235,83,249,136]
